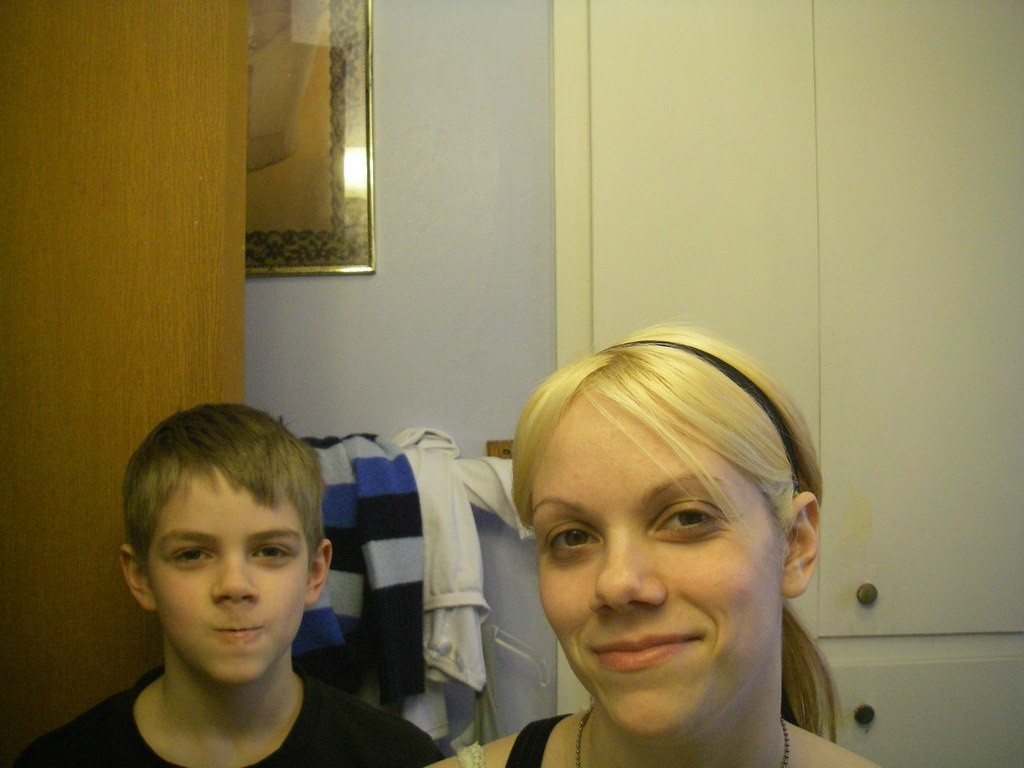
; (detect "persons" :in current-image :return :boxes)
[419,324,884,768]
[9,402,444,768]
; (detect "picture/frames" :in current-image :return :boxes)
[247,1,377,275]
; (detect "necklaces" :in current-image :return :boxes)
[575,707,789,768]
